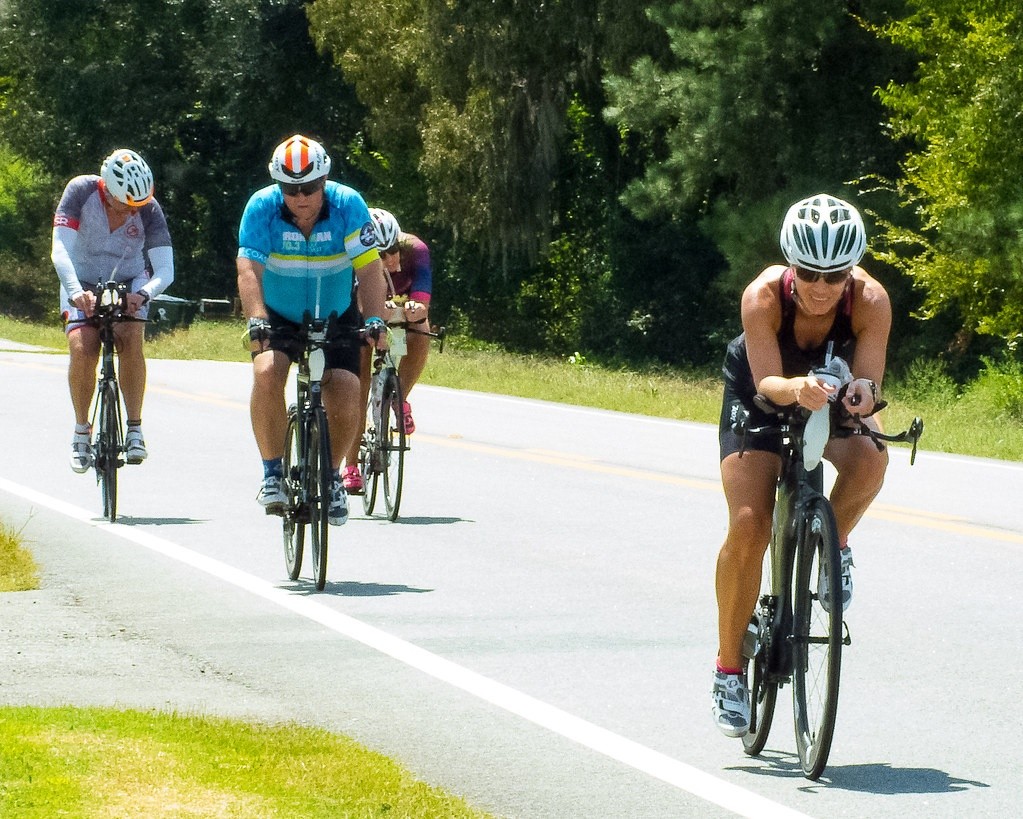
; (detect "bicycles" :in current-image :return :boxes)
[65,278,162,522]
[248,308,387,592]
[343,314,448,523]
[734,374,925,781]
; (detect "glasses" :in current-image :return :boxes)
[795,265,851,285]
[378,239,399,259]
[278,175,325,196]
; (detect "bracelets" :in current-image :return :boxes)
[869,380,878,402]
[247,316,269,327]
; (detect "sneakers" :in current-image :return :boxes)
[328,475,349,526]
[391,399,415,435]
[70,431,92,474]
[126,431,148,459]
[256,475,288,507]
[817,534,855,613]
[711,648,751,738]
[341,466,363,490]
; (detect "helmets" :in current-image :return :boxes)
[269,135,331,184]
[100,149,154,206]
[367,208,401,253]
[779,194,866,273]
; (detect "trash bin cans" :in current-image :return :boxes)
[143,299,197,342]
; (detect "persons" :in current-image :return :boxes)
[708,193,891,738]
[52,148,175,474]
[237,134,434,526]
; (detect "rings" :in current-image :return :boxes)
[364,316,385,327]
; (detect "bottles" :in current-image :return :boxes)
[308,319,326,382]
[390,300,408,356]
[371,373,384,426]
[802,370,841,472]
[101,281,118,305]
